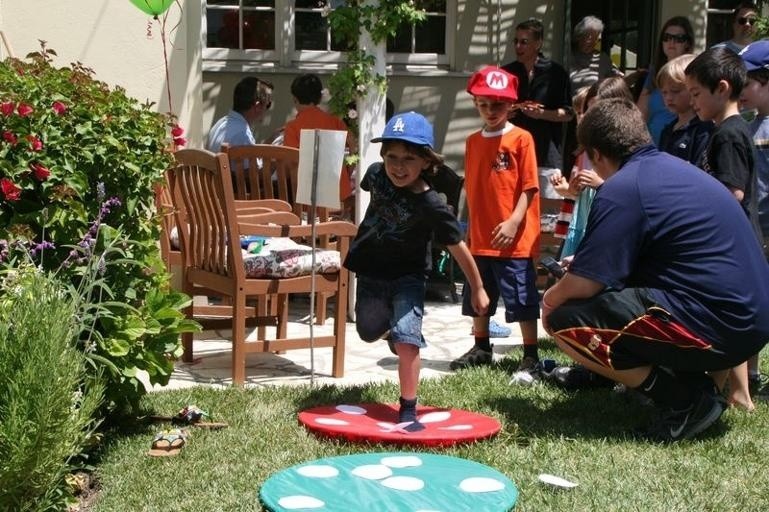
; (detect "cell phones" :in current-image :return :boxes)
[539,254,564,279]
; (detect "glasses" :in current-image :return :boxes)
[661,32,689,44]
[737,17,757,26]
[513,38,535,46]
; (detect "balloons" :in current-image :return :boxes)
[128,1,174,20]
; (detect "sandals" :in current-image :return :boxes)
[471,319,511,338]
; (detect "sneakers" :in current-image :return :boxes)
[749,372,769,400]
[450,343,494,370]
[515,355,542,376]
[652,388,724,444]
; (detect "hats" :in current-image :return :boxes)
[466,63,520,103]
[369,109,435,151]
[738,40,769,74]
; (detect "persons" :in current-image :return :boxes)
[451,66,546,384]
[343,109,489,431]
[489,6,769,444]
[206,75,271,195]
[285,71,356,219]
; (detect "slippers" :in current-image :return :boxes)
[147,426,187,459]
[149,404,229,429]
